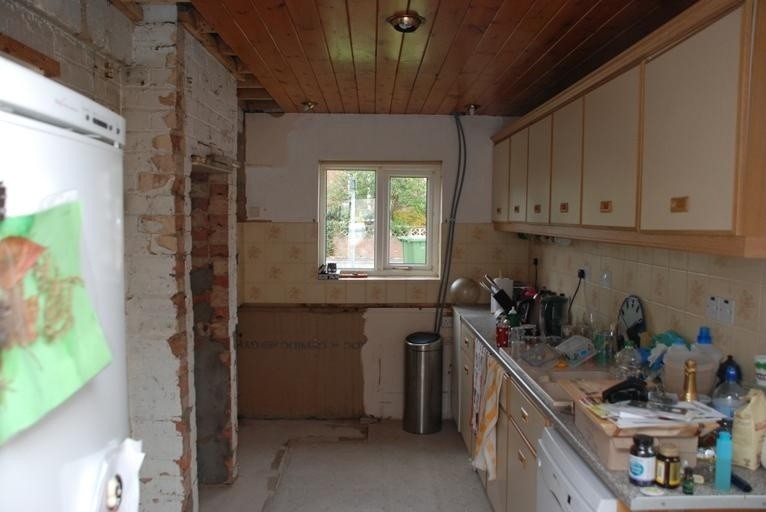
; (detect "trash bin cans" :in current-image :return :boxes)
[404,333,442,434]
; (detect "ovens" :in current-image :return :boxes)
[527,424,616,512]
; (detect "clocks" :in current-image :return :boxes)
[615,294,645,345]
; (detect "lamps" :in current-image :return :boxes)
[387,0,426,34]
[302,101,318,110]
[467,104,479,115]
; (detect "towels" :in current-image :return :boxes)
[471,336,505,482]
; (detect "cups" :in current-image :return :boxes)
[595,329,612,362]
[752,355,766,389]
[564,310,599,338]
[507,325,546,364]
[496,326,508,347]
[664,351,714,392]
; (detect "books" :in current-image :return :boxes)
[579,397,736,428]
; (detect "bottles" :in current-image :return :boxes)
[714,367,742,418]
[692,328,723,363]
[715,430,734,493]
[615,340,641,382]
[508,306,521,327]
[714,356,742,387]
[682,466,694,494]
[668,338,691,353]
[627,434,656,486]
[681,360,699,401]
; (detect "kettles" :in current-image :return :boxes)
[539,295,569,339]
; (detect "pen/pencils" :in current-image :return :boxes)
[730,472,753,493]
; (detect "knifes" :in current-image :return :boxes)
[479,275,501,295]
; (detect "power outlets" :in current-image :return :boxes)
[576,266,590,282]
[531,255,541,267]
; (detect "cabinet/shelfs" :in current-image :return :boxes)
[508,374,551,511]
[472,335,508,511]
[582,0,766,259]
[528,77,585,239]
[490,111,529,233]
[450,307,475,454]
